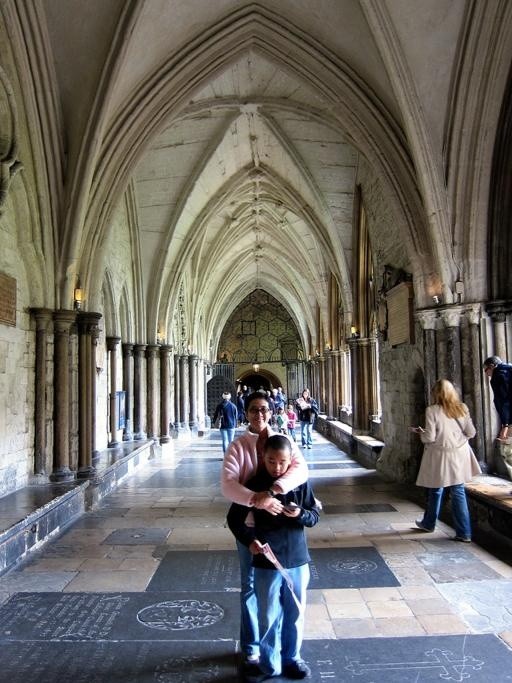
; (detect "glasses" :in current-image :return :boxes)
[245,406,270,415]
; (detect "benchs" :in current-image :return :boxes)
[312,407,512,556]
[0,415,199,580]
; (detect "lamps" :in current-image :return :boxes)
[73,270,85,311]
[350,326,361,337]
[157,322,162,339]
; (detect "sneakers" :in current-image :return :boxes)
[303,443,312,449]
[415,518,435,532]
[239,654,282,682]
[450,532,471,543]
[282,659,311,680]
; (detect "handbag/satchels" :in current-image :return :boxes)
[211,416,221,428]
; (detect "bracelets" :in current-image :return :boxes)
[267,489,275,497]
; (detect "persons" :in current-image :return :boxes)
[484,356,512,482]
[219,392,309,666]
[226,435,320,683]
[409,379,482,542]
[213,384,318,454]
